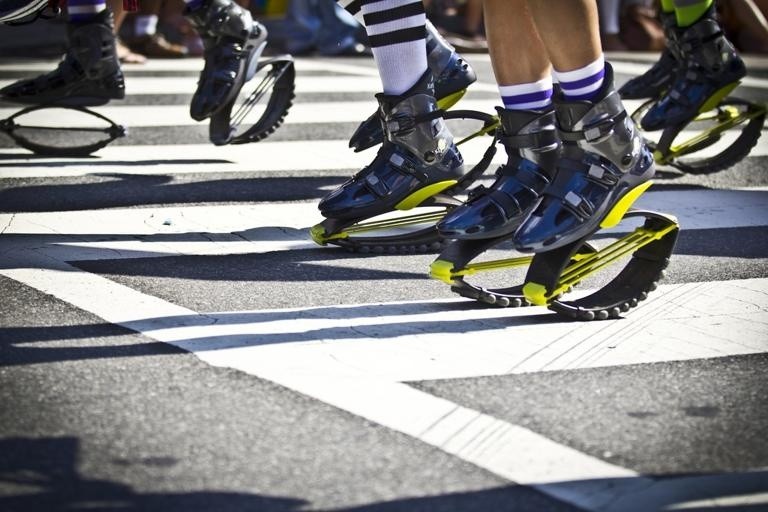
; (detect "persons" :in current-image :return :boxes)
[306,1,479,221]
[432,0,656,255]
[0,0,269,122]
[615,0,749,134]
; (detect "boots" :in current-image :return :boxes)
[181,0,270,122]
[617,0,748,132]
[318,17,480,222]
[0,6,127,103]
